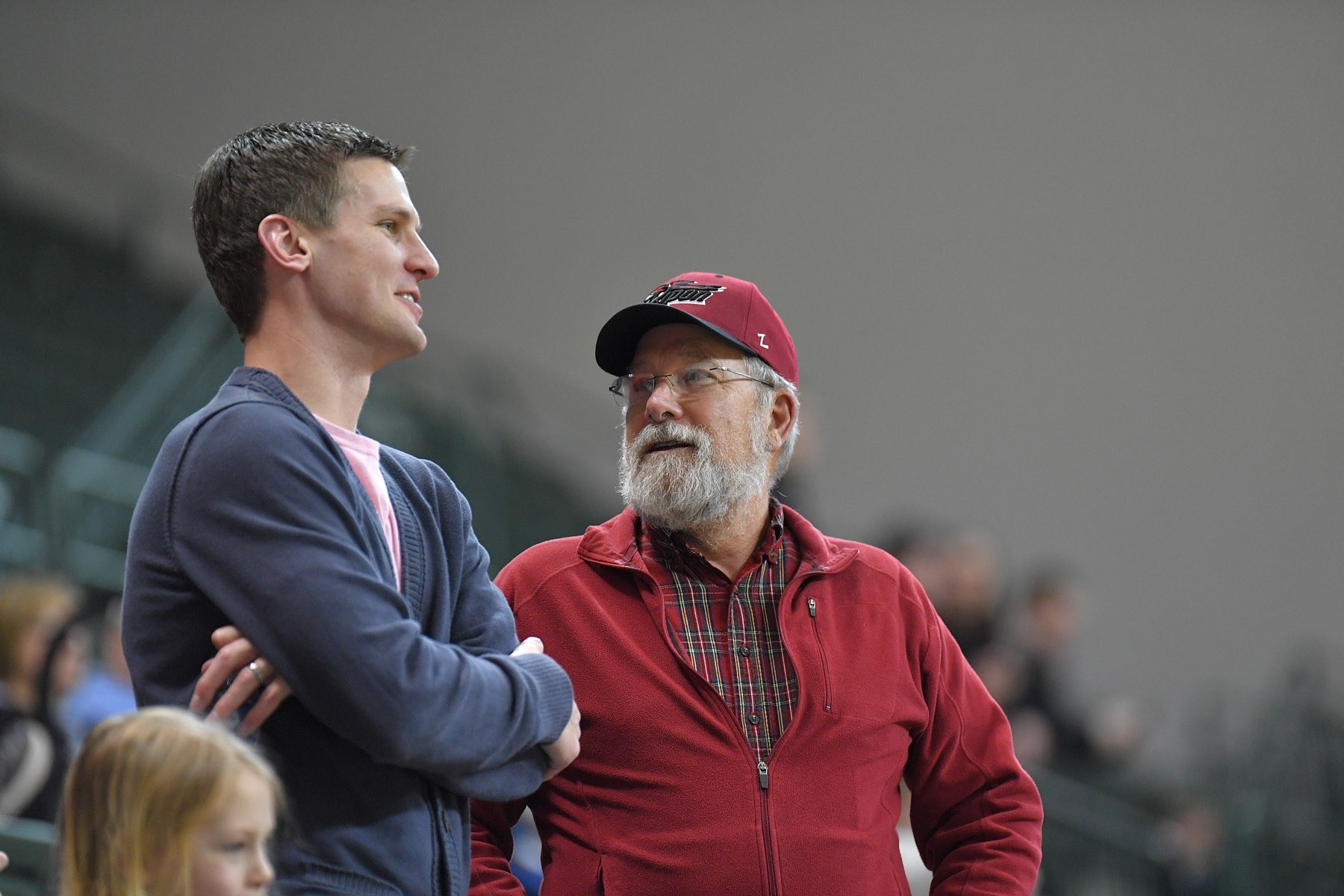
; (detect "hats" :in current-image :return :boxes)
[594,272,798,394]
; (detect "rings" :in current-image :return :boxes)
[250,661,264,688]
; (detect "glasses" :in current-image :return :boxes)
[609,363,777,407]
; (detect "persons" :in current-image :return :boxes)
[124,121,581,895]
[55,705,286,895]
[469,270,1044,896]
[0,524,1230,896]
